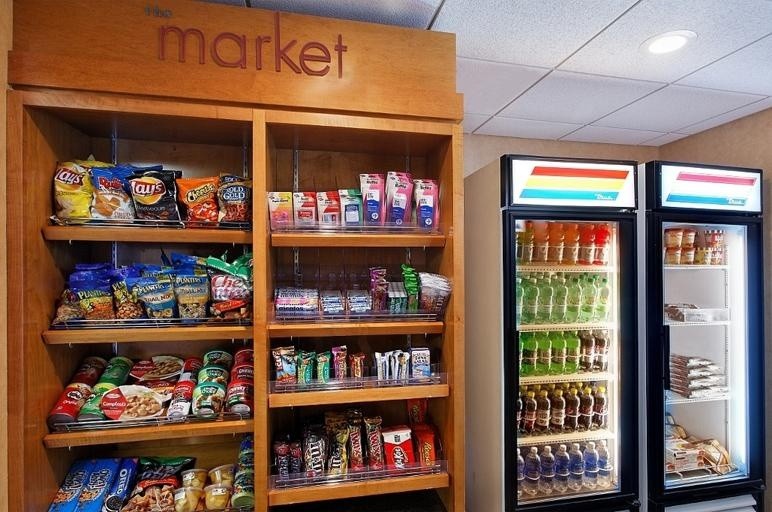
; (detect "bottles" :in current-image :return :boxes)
[509,217,613,265]
[513,438,611,497]
[516,329,609,377]
[516,383,606,430]
[511,269,608,326]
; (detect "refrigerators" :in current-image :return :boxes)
[637,161,767,512]
[463,155,639,512]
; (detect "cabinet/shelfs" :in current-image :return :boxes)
[5,86,467,512]
